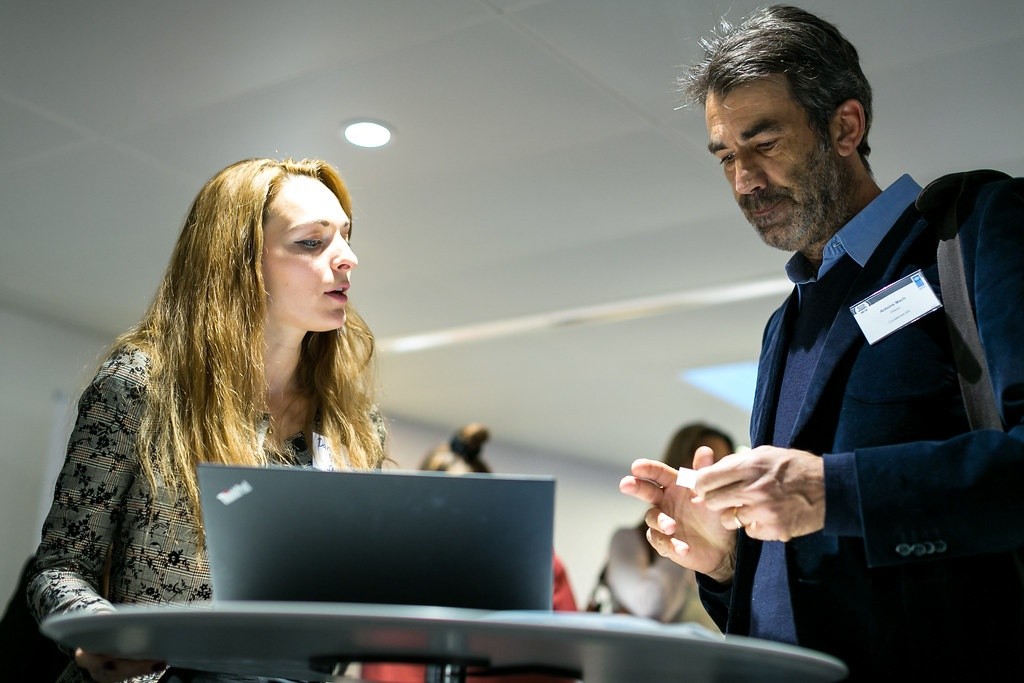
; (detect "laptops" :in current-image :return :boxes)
[195,460,555,607]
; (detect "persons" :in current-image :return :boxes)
[22,156,384,683]
[362,424,584,682]
[617,9,1024,683]
[604,424,745,627]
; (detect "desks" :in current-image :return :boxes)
[40,602,849,683]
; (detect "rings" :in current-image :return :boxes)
[731,506,744,528]
